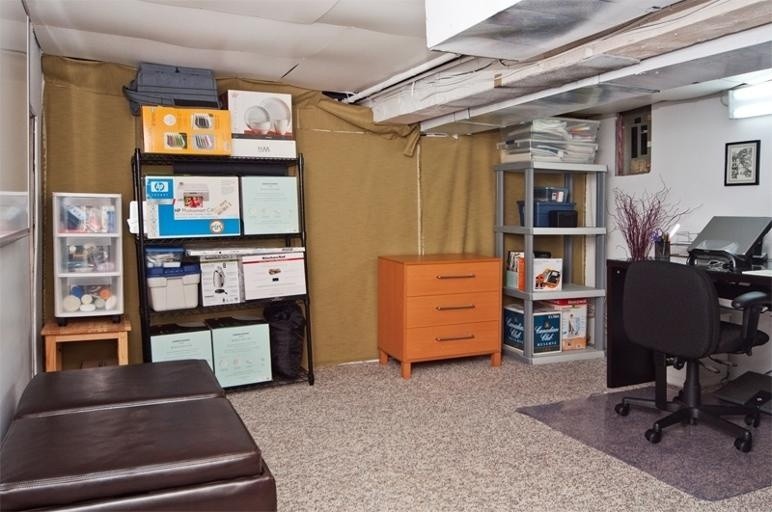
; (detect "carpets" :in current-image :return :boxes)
[513,384,772,500]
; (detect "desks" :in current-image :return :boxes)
[606,257,772,419]
[41,313,132,373]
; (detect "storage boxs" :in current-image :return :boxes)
[142,320,213,375]
[145,263,201,313]
[240,174,301,241]
[240,251,309,305]
[504,186,596,358]
[496,116,601,163]
[221,90,301,160]
[140,171,244,241]
[136,104,235,159]
[197,254,246,308]
[145,246,185,270]
[212,313,272,389]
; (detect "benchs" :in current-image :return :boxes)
[0,358,280,512]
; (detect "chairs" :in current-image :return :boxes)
[611,260,760,453]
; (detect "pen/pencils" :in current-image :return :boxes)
[667,223,681,242]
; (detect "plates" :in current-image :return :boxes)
[245,97,292,133]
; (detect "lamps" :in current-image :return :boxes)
[728,79,772,119]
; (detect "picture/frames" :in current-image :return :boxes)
[723,139,760,186]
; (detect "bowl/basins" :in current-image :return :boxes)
[250,121,272,136]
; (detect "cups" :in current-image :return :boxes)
[274,118,291,136]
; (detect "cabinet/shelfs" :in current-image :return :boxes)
[491,159,611,367]
[51,192,126,326]
[376,250,504,380]
[130,146,316,391]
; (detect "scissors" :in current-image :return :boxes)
[650,227,664,243]
